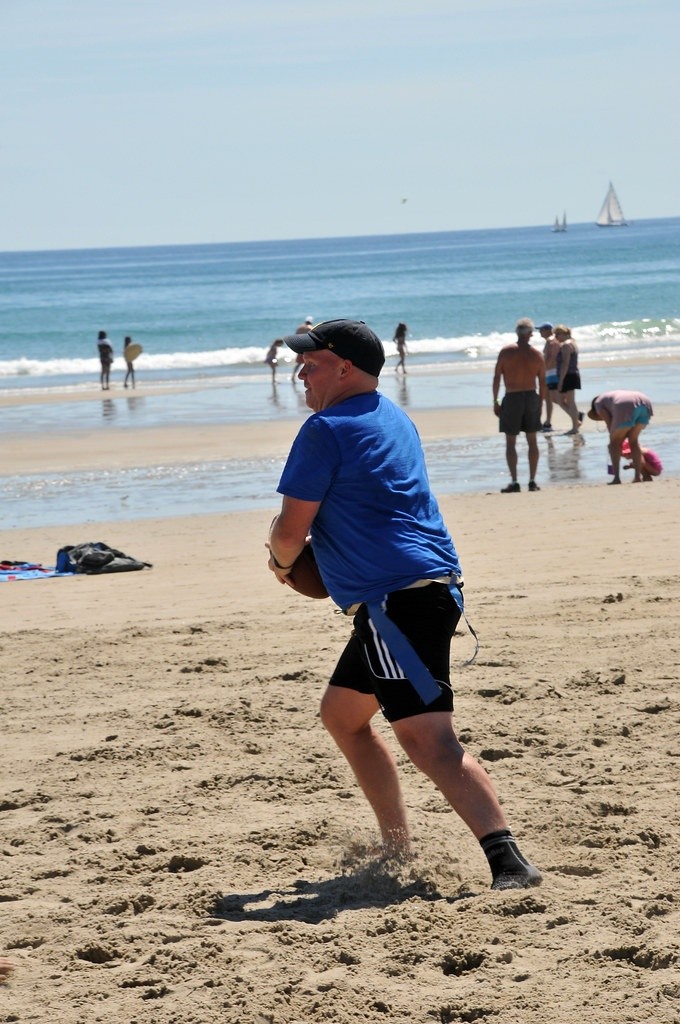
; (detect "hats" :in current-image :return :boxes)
[304,316,314,323]
[619,439,639,456]
[282,318,385,378]
[534,323,553,330]
[587,395,606,421]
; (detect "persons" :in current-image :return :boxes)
[393,322,408,373]
[290,315,314,385]
[264,318,543,896]
[124,337,134,386]
[589,390,653,483]
[493,318,546,493]
[621,438,662,481]
[97,331,113,390]
[554,325,582,435]
[265,339,283,378]
[534,323,585,431]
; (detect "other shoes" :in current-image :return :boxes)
[562,429,581,436]
[539,421,553,430]
[528,480,540,491]
[578,411,585,423]
[501,482,521,492]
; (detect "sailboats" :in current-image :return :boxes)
[550,209,568,232]
[595,180,628,227]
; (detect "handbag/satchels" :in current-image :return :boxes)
[56,541,153,574]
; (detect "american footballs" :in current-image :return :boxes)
[269,513,332,600]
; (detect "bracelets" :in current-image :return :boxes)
[494,400,498,404]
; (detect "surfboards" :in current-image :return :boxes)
[123,344,142,362]
